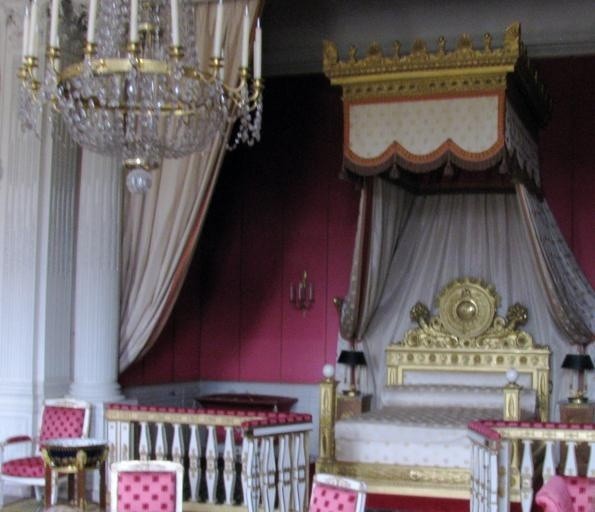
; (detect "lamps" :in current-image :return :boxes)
[18,0,266,195]
[560,354,595,405]
[336,350,368,396]
[287,269,316,311]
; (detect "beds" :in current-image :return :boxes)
[320,277,552,503]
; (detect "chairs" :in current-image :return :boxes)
[0,398,91,506]
[307,474,368,511]
[108,461,184,512]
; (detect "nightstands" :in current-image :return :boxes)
[337,393,372,418]
[559,402,595,427]
